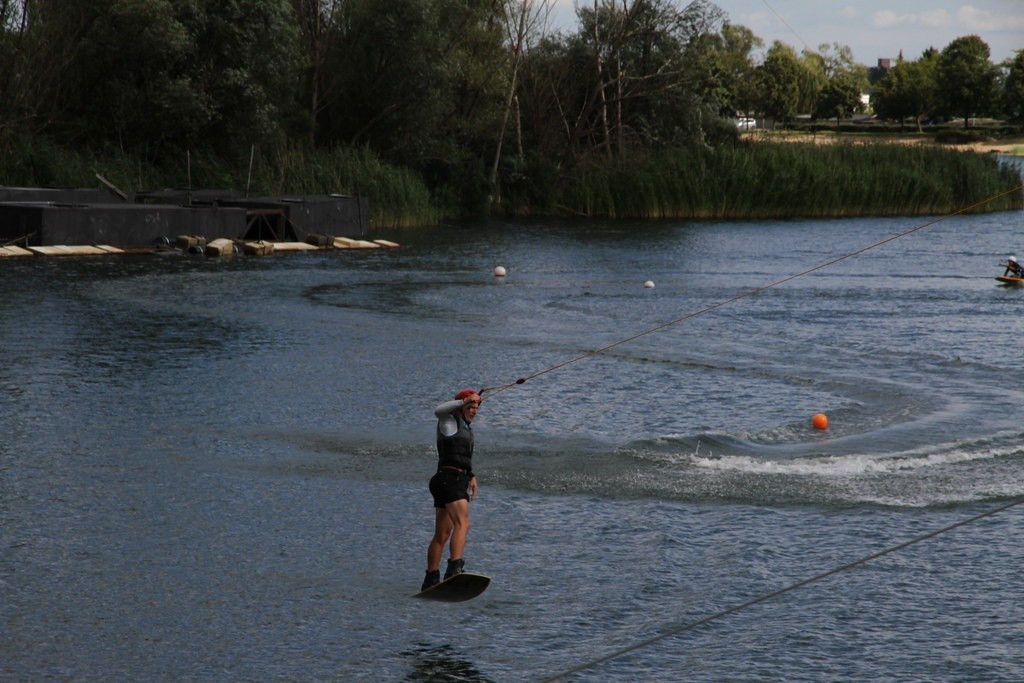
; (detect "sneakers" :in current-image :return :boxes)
[443,559,465,580]
[421,569,440,591]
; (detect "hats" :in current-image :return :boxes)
[1007,255,1017,263]
[455,389,481,407]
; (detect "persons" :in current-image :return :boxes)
[1004,256,1024,278]
[420,389,482,591]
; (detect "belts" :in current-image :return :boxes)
[441,465,464,472]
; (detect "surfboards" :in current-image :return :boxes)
[409,572,492,603]
